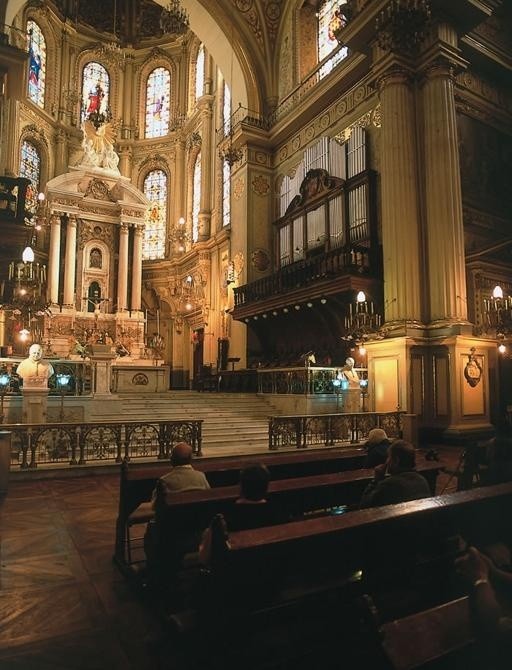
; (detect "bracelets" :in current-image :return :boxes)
[465,578,490,594]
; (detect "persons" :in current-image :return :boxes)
[142,443,215,569]
[456,415,512,491]
[14,341,57,387]
[101,140,121,171]
[340,356,360,388]
[80,138,98,167]
[221,460,293,533]
[452,545,512,643]
[359,428,392,470]
[84,297,106,330]
[356,442,438,511]
